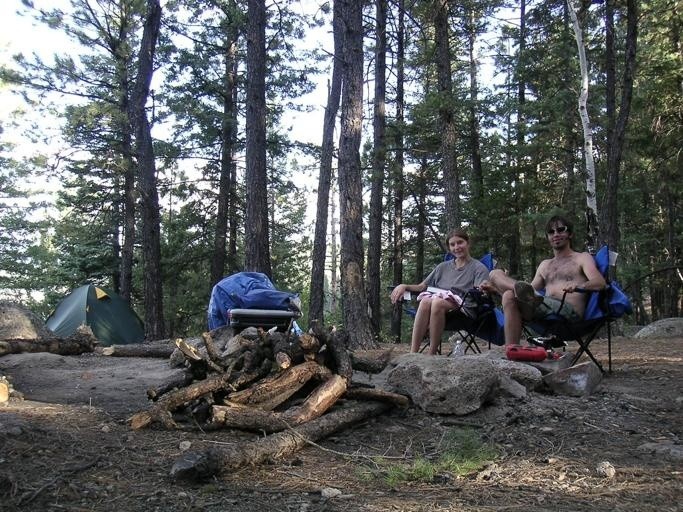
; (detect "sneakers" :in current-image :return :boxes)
[513,280,544,309]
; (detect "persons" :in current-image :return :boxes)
[390,229,490,355]
[488,214,607,348]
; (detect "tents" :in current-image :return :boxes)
[44,284,145,346]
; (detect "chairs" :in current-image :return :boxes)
[397,245,632,378]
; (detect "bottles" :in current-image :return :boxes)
[452,339,464,358]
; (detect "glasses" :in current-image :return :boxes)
[547,225,569,234]
